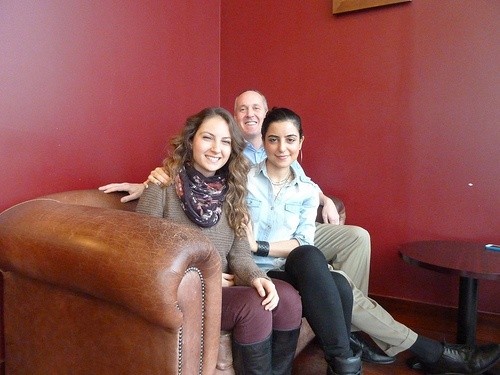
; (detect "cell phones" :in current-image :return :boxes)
[485,244,500,251]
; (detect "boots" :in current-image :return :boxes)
[231,331,272,375]
[324,346,362,375]
[272,319,303,375]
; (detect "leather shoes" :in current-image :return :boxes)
[350,332,395,364]
[407,340,500,375]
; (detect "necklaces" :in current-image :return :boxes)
[271,169,292,185]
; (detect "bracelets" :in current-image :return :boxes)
[253,241,270,257]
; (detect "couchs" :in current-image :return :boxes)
[0,190,346,375]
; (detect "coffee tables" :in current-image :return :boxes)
[400,240,500,375]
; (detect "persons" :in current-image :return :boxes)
[99,107,363,375]
[233,90,500,375]
[138,107,302,375]
